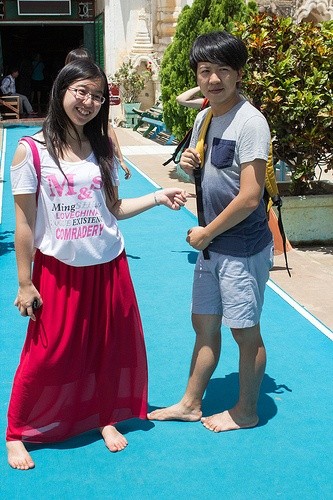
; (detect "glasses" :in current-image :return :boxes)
[67,85,106,104]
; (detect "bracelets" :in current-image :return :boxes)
[154,192,160,206]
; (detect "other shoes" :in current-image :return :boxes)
[22,112,26,117]
[29,111,38,115]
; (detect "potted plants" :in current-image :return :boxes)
[114,61,153,128]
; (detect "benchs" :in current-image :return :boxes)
[131,98,178,145]
[0,96,23,119]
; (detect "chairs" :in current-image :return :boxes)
[233,11,333,248]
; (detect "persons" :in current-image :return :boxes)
[4,58,198,470]
[147,31,271,433]
[0,68,38,115]
[30,50,45,113]
[65,48,90,64]
